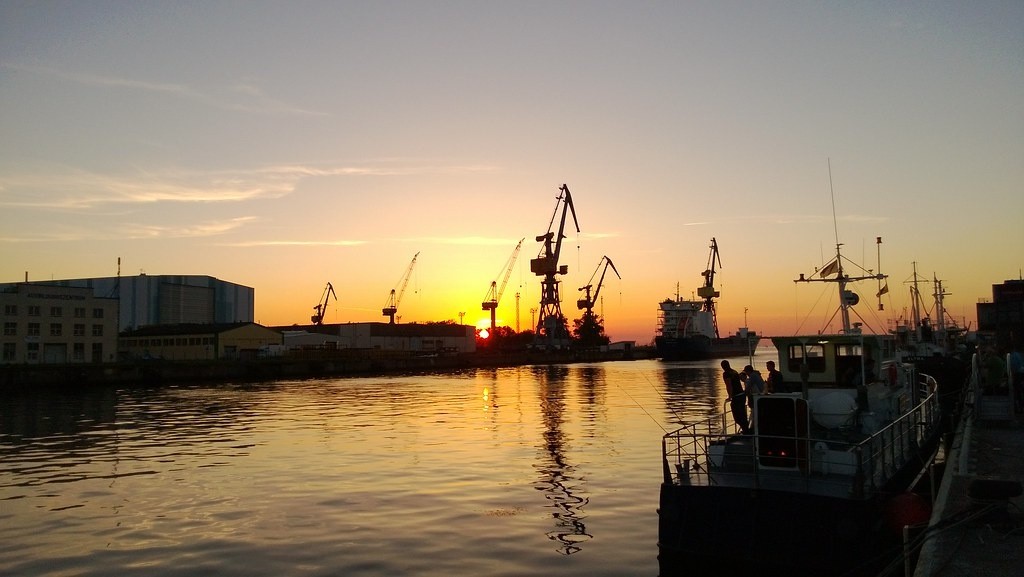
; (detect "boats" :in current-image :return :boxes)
[655,244,983,577]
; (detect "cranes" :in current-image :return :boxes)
[696,237,724,339]
[482,238,526,328]
[530,183,581,337]
[577,257,623,338]
[310,281,339,324]
[381,250,422,325]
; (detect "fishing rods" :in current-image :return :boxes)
[614,381,719,485]
[636,365,718,470]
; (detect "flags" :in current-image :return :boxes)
[876,283,889,297]
[820,259,838,277]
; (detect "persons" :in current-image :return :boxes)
[721,360,751,438]
[765,360,782,393]
[739,372,753,408]
[743,365,767,429]
